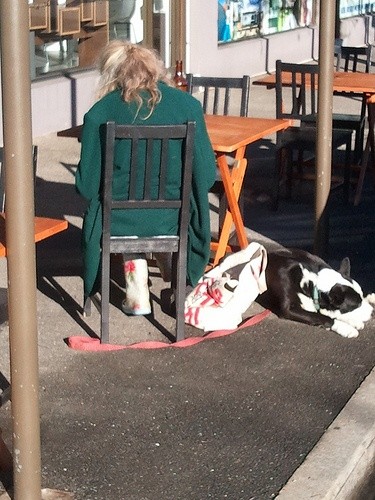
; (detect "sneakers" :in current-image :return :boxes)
[122,299,151,315]
[170,292,177,305]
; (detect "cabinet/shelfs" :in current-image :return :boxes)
[28,0,112,71]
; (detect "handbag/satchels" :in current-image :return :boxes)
[184,242,267,332]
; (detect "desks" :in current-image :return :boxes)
[252,72,375,204]
[58,115,290,270]
[0,213,68,259]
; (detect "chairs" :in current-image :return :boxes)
[272,59,354,211]
[185,74,251,245]
[332,45,371,177]
[0,144,38,215]
[83,120,196,346]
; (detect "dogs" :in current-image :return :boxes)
[214,230,374,338]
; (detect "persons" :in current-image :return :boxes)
[76,39,217,315]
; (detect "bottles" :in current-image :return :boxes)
[172,60,188,93]
